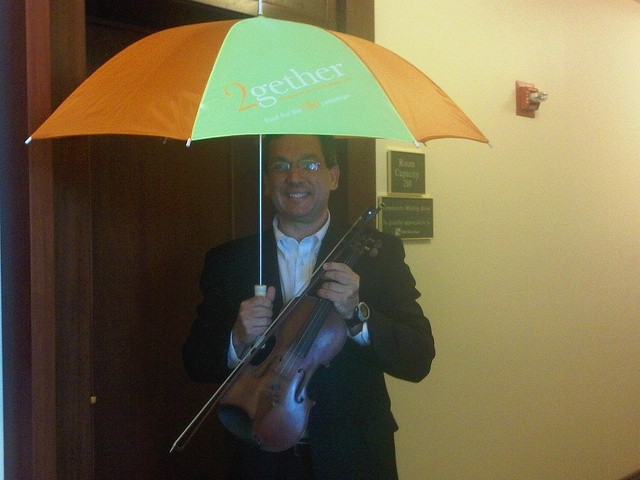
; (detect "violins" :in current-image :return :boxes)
[216,232,382,453]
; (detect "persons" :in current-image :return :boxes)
[194,134,435,476]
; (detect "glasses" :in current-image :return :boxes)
[266,159,327,173]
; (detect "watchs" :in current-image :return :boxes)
[344,301,369,326]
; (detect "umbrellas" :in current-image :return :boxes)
[24,0,492,350]
